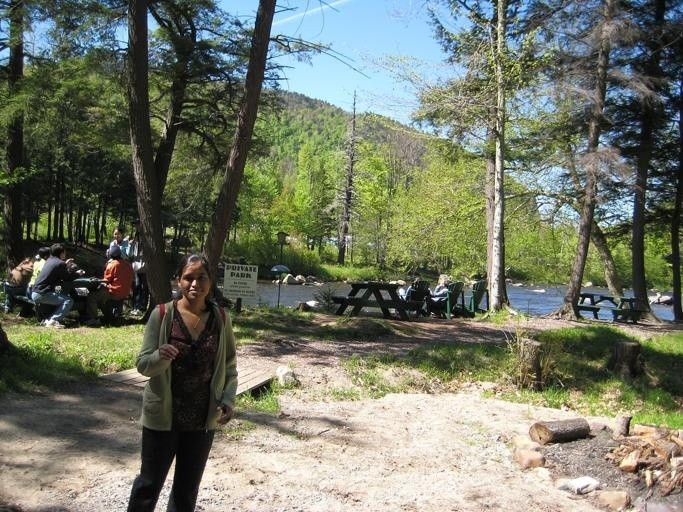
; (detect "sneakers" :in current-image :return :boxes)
[45,319,67,330]
[129,309,142,315]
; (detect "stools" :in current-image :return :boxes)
[4,278,123,323]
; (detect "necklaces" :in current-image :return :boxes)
[180,299,207,329]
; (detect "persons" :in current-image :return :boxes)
[395,274,455,318]
[3,217,150,329]
[120,252,237,510]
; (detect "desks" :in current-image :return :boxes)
[331,282,409,322]
[578,293,641,325]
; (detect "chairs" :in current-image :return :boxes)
[400,280,489,320]
